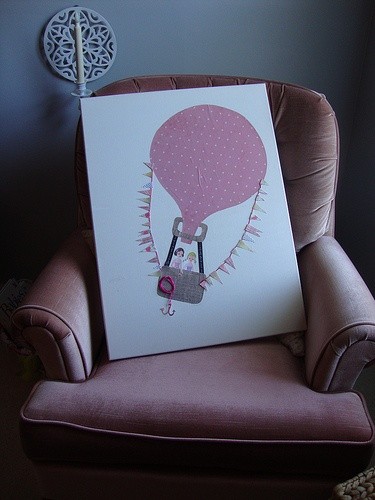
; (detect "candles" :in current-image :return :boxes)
[75,12,84,83]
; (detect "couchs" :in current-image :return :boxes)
[8,73,375,500]
